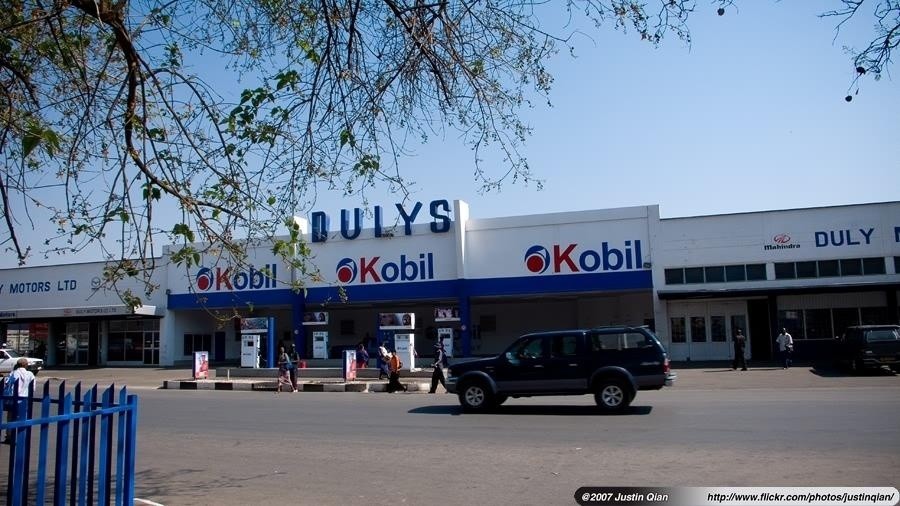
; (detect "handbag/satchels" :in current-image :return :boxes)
[398,361,403,369]
[285,362,293,370]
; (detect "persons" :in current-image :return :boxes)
[428,342,448,393]
[376,340,407,393]
[37,340,46,359]
[440,335,447,364]
[732,330,748,371]
[776,328,794,370]
[3,358,36,444]
[276,339,300,393]
[357,343,370,368]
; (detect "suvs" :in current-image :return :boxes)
[443,324,676,411]
[823,323,900,376]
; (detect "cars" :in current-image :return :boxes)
[0,348,44,377]
